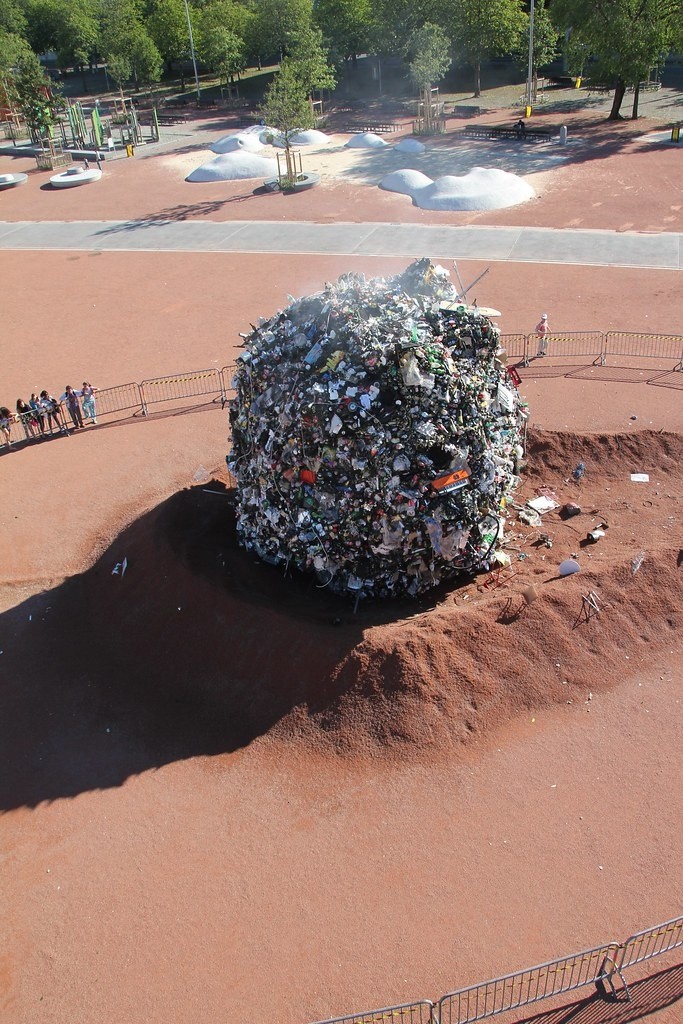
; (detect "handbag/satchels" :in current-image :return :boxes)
[48,399,61,413]
[30,416,38,426]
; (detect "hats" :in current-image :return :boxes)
[542,314,547,319]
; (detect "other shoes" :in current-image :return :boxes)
[93,421,97,424]
[537,354,541,356]
[540,352,546,355]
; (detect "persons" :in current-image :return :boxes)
[535,313,552,357]
[517,119,526,140]
[39,390,66,437]
[0,407,20,451]
[59,385,85,429]
[16,398,39,442]
[29,393,47,438]
[81,381,100,425]
